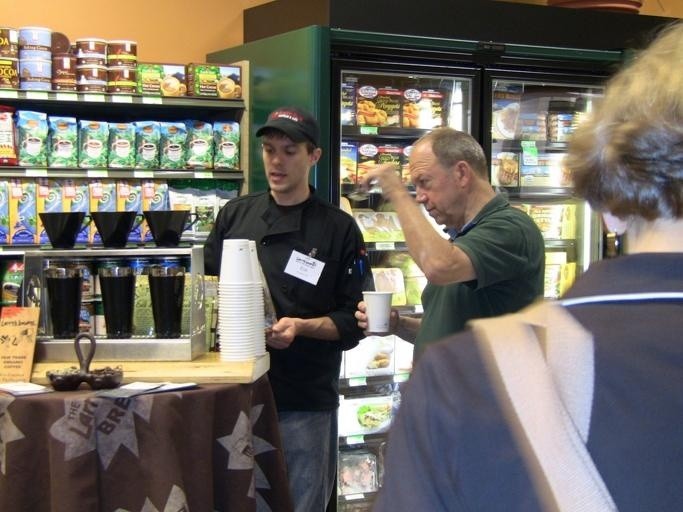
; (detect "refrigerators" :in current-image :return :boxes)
[205,21,480,511]
[482,35,625,296]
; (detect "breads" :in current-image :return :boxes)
[366,352,389,369]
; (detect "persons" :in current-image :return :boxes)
[373,12,682,512]
[201,107,377,511]
[359,117,548,372]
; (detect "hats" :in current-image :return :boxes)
[256,105,318,141]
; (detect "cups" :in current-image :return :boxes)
[141,210,200,249]
[44,267,85,338]
[89,210,145,250]
[37,211,92,248]
[147,267,186,337]
[217,236,267,361]
[362,289,394,333]
[99,265,136,337]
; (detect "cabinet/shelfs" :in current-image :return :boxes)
[0,60,252,341]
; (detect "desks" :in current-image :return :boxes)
[0,352,294,512]
